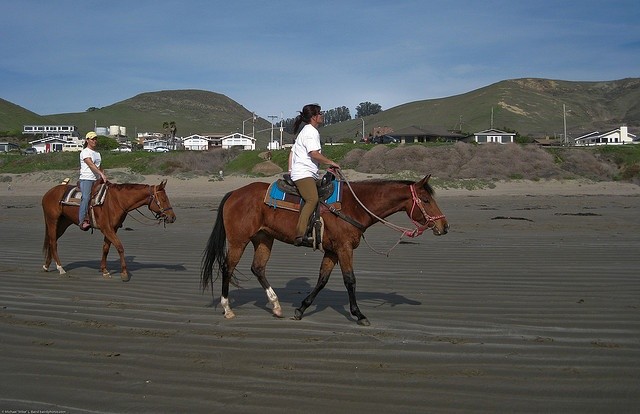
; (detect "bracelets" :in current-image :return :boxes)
[289,169,292,172]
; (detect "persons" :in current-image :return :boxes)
[288,103,341,246]
[79,131,107,230]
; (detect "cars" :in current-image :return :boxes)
[111,145,132,152]
[372,134,400,144]
[25,147,36,153]
[148,147,169,152]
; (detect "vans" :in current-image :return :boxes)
[62,144,84,152]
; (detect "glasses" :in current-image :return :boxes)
[316,112,322,115]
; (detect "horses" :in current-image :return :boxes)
[198,175,450,326]
[41,179,176,282]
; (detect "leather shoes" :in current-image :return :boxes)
[294,237,314,246]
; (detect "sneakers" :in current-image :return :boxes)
[82,222,90,228]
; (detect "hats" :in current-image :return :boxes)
[85,131,98,140]
